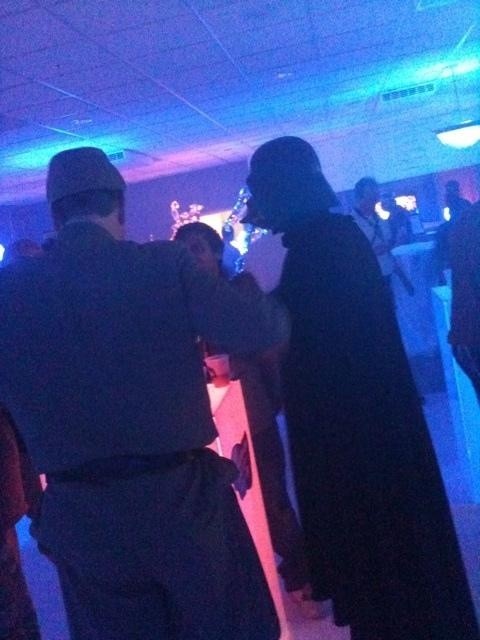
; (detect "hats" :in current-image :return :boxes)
[248,135,341,213]
[45,144,126,205]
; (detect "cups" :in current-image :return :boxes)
[204,353,231,388]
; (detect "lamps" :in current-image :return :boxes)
[432,78,479,149]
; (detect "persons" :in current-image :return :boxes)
[446,197,480,409]
[219,224,241,275]
[380,187,412,247]
[439,179,472,222]
[1,406,58,640]
[240,134,480,640]
[349,176,426,406]
[173,221,326,620]
[0,145,292,640]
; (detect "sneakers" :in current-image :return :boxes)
[288,582,329,620]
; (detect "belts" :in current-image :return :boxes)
[53,448,203,480]
